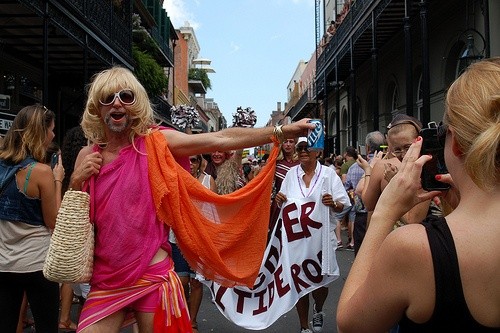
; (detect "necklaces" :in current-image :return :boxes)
[297,165,322,198]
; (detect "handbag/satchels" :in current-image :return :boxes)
[43,144,100,284]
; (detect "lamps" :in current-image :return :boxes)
[458,31,484,67]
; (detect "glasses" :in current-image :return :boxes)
[392,148,409,157]
[97,88,136,105]
[41,106,49,118]
[189,158,197,163]
[296,146,310,153]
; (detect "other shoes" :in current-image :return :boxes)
[337,242,343,248]
[345,244,354,251]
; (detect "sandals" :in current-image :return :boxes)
[23,319,34,329]
[58,320,76,333]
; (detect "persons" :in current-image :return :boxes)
[324,114,460,256]
[169,139,300,333]
[0,105,88,333]
[276,136,351,333]
[67,67,326,333]
[335,59,500,333]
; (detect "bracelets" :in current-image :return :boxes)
[366,175,370,176]
[273,125,284,142]
[54,180,63,184]
[334,201,337,205]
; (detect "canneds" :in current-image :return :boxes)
[307,119,324,152]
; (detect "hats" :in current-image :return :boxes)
[295,137,307,146]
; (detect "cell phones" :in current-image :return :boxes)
[359,145,368,161]
[50,153,58,170]
[418,128,451,190]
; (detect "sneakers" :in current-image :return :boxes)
[312,302,325,332]
